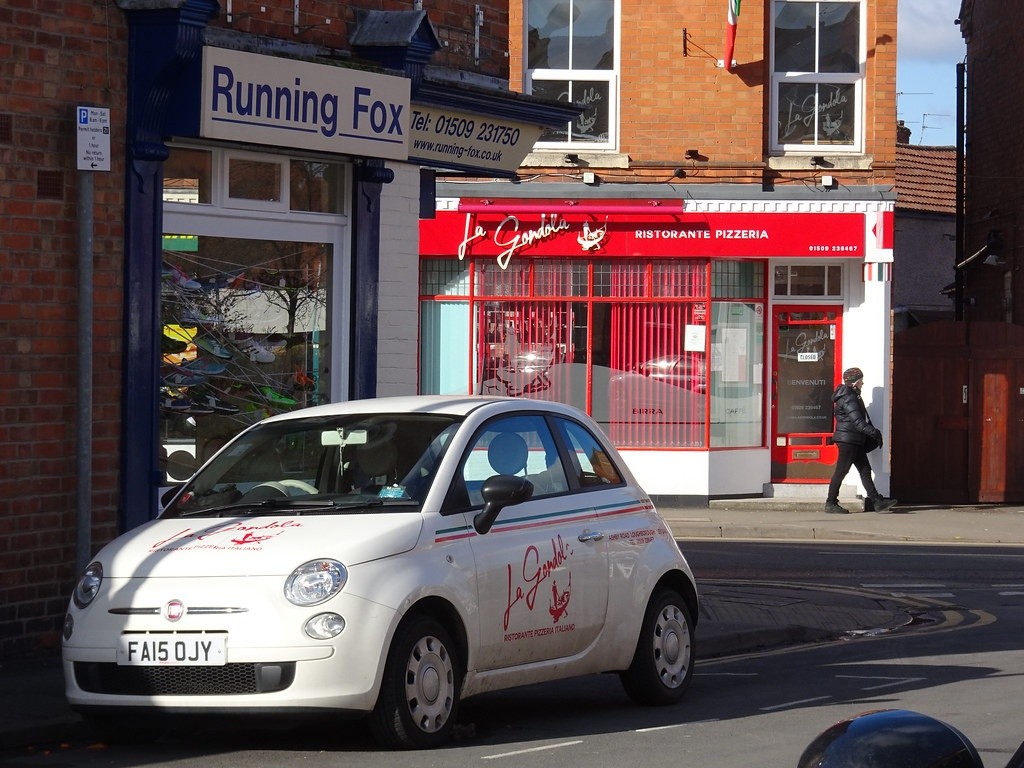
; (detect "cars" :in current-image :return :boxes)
[55,397,704,745]
[613,355,712,417]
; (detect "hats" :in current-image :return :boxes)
[843,368,863,383]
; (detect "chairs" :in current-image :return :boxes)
[340,435,397,496]
[488,433,544,496]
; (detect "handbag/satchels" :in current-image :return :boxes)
[865,407,878,452]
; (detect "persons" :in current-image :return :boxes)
[822,368,900,517]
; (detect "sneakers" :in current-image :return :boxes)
[825,502,849,514]
[874,498,897,512]
